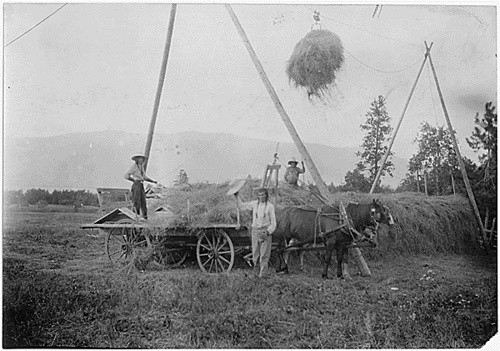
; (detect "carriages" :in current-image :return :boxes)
[82,197,394,278]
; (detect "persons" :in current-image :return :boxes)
[124,154,157,219]
[284,161,305,186]
[233,188,276,278]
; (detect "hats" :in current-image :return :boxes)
[226,180,245,196]
[287,157,299,165]
[130,148,148,160]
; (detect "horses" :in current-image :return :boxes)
[278,199,395,279]
